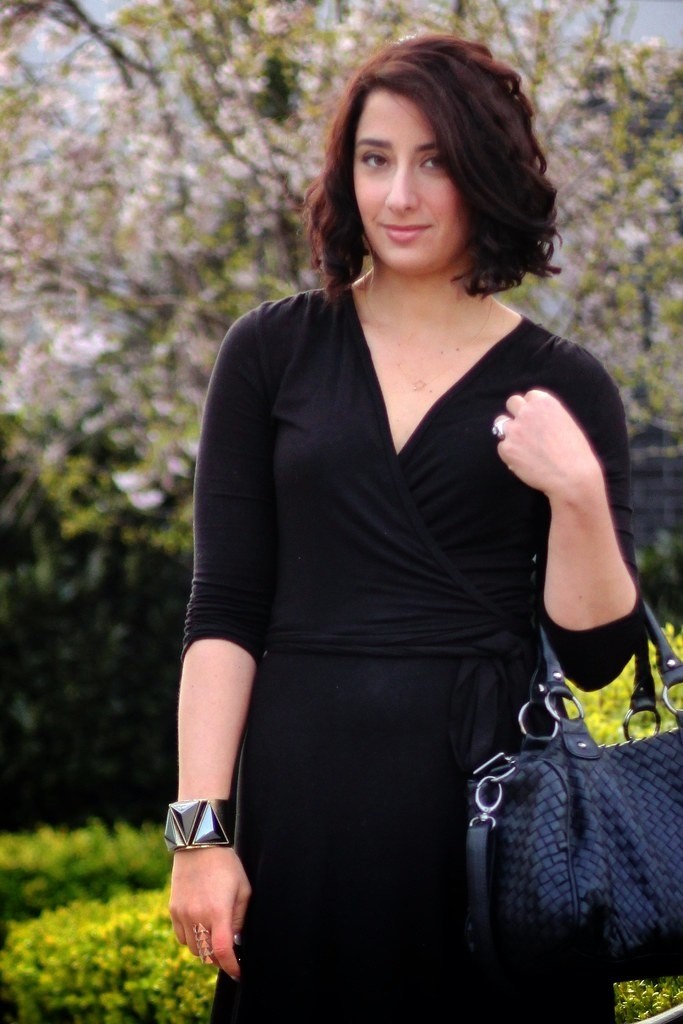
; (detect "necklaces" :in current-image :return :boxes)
[363,274,493,390]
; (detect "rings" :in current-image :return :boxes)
[492,418,511,440]
[194,923,214,964]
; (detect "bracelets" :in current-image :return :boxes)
[163,799,233,852]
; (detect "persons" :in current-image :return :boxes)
[167,35,644,1024]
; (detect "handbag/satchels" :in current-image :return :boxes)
[468,595,683,983]
